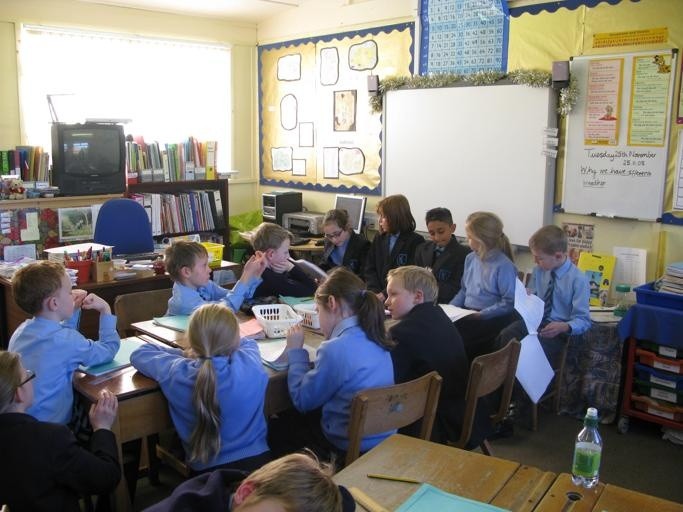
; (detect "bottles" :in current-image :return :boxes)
[572,407,604,488]
[614,283,631,317]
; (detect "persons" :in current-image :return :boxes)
[285,267,397,467]
[239,222,319,316]
[140,453,355,512]
[322,186,517,355]
[8,261,120,423]
[385,265,484,441]
[0,351,122,512]
[162,240,267,315]
[129,302,269,473]
[494,225,591,422]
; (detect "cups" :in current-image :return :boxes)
[67,259,92,285]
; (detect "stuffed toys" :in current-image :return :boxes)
[0,179,28,199]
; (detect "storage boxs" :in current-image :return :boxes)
[633,284,683,310]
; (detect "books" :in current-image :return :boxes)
[288,256,327,278]
[239,319,317,370]
[0,188,224,282]
[577,250,618,307]
[78,315,188,377]
[653,262,683,296]
[125,133,206,184]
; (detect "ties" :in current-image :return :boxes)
[538,273,554,333]
[434,247,442,265]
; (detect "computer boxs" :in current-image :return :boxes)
[262,190,302,226]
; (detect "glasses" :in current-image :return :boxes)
[10,367,36,404]
[323,229,344,239]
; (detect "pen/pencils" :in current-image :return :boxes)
[60,246,93,262]
[366,474,421,485]
[96,247,113,262]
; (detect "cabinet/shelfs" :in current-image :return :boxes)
[0,194,126,260]
[125,179,229,260]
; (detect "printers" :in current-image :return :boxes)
[283,212,325,235]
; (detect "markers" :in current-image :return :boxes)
[589,212,614,219]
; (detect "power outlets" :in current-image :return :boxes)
[368,218,381,231]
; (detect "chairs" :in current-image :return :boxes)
[444,337,521,456]
[514,273,571,431]
[92,198,153,255]
[114,289,173,339]
[344,371,443,468]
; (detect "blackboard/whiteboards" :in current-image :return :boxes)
[557,48,679,222]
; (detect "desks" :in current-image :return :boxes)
[563,304,628,425]
[74,335,191,512]
[0,260,242,342]
[330,433,683,512]
[130,308,401,419]
[288,237,325,260]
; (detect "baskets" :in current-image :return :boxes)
[251,304,303,339]
[293,301,321,330]
[201,242,225,270]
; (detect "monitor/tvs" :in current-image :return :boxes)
[50,121,127,195]
[333,194,368,235]
